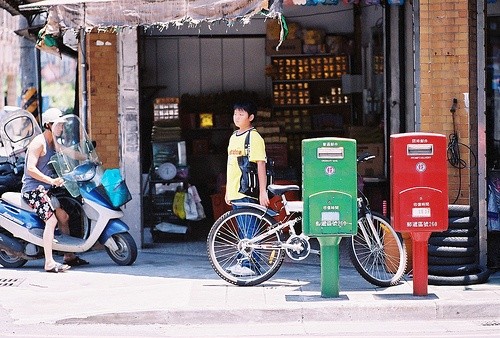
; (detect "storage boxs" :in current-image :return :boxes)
[356,141,385,178]
[333,113,344,129]
[153,142,179,164]
[265,39,302,55]
[302,29,325,45]
[191,136,209,156]
[265,18,301,39]
[320,112,333,130]
[312,114,321,129]
[302,44,326,54]
[153,97,179,122]
[327,36,346,53]
[346,126,384,142]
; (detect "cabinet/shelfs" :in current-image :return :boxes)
[183,125,235,243]
[148,164,190,244]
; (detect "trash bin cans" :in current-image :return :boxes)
[382,223,413,273]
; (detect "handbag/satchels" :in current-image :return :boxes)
[237,129,275,200]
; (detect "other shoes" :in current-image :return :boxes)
[231,266,256,276]
[226,264,242,273]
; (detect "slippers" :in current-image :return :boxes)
[63,256,89,265]
[46,262,71,272]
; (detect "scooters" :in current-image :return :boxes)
[0,106,43,197]
[0,113,138,269]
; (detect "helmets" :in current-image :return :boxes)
[42,108,68,126]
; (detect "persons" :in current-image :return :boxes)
[224,101,270,277]
[21,107,101,273]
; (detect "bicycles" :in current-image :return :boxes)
[206,184,409,287]
